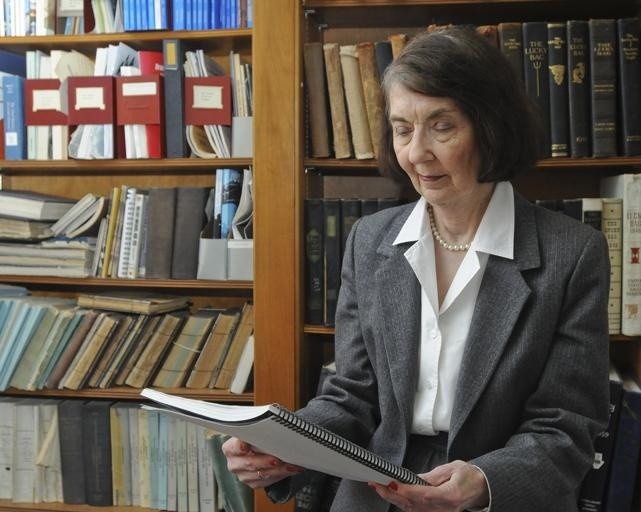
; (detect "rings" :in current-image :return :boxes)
[258,471,271,480]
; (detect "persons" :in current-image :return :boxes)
[224,22,609,512]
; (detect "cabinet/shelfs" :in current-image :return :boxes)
[0,0,641,512]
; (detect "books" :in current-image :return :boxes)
[302,18,640,160]
[1,285,254,394]
[25,43,165,160]
[183,50,253,159]
[2,0,252,37]
[1,397,254,512]
[1,166,254,280]
[305,175,641,337]
[293,363,341,512]
[577,350,641,512]
[139,389,435,488]
